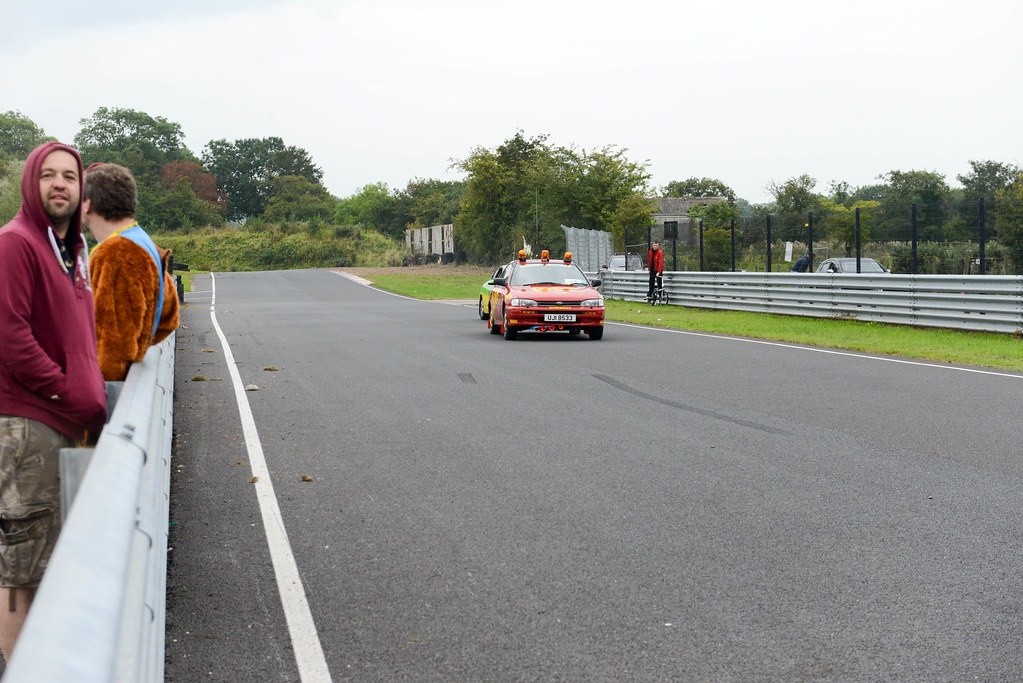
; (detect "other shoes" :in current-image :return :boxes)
[643,296,654,300]
[657,295,664,300]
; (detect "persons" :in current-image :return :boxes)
[647,240,664,296]
[82,162,181,382]
[791,252,816,287]
[0,142,108,662]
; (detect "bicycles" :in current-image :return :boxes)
[644,275,673,307]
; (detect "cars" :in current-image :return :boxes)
[479,265,507,320]
[488,250,606,340]
[814,258,893,292]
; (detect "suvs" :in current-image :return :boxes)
[602,252,647,273]
